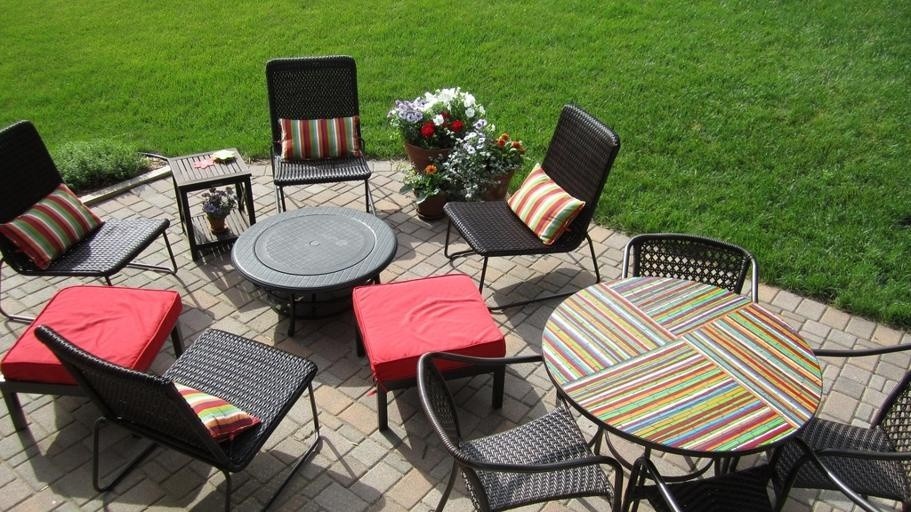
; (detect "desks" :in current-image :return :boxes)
[542,277,825,476]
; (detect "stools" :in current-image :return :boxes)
[0,285,186,433]
[354,273,506,431]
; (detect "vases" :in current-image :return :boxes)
[402,142,457,174]
[410,188,451,219]
[206,213,228,234]
[477,170,515,201]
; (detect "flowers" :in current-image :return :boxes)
[397,160,463,205]
[386,87,486,149]
[202,186,239,216]
[439,120,527,202]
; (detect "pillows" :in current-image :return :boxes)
[277,115,362,160]
[172,382,263,443]
[2,183,104,270]
[507,162,586,245]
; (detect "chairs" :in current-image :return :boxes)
[621,435,882,512]
[768,343,911,512]
[1,120,178,321]
[265,55,378,214]
[622,232,758,304]
[444,105,621,310]
[34,324,321,512]
[416,350,624,511]
